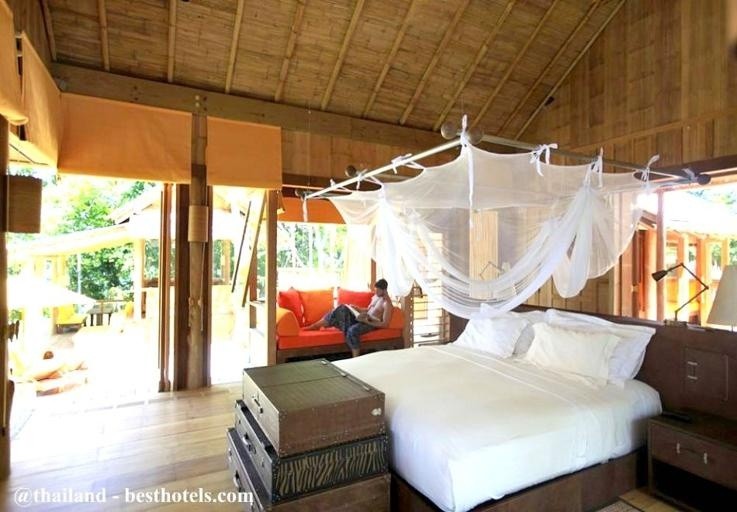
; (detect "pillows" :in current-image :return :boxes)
[454,304,658,390]
[276,287,376,328]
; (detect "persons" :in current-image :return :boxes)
[304,279,393,358]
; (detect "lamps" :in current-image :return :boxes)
[652,262,737,333]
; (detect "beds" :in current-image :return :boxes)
[329,303,685,511]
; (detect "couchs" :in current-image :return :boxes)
[276,293,404,359]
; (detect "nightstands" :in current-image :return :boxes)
[643,409,737,512]
